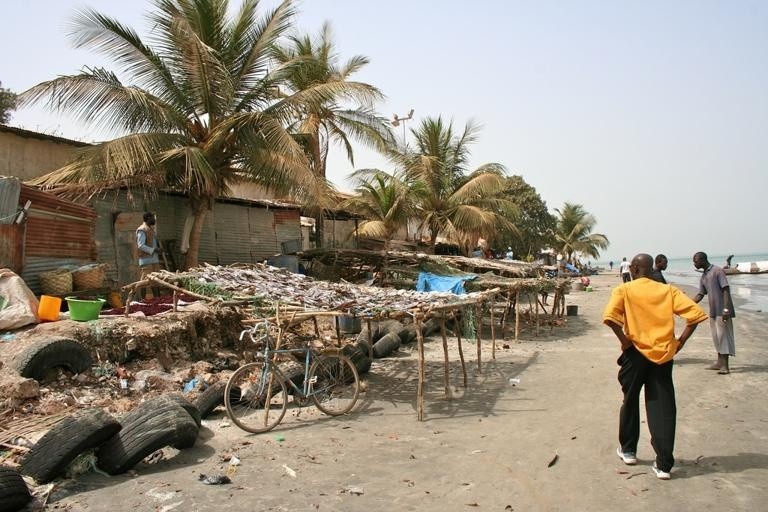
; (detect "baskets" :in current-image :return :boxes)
[71,262,108,291]
[38,268,74,295]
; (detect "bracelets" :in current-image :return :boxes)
[723,308,730,313]
[723,304,730,308]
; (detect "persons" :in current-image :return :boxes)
[560,256,591,275]
[133,211,163,298]
[620,257,631,283]
[470,245,521,276]
[652,254,668,284]
[726,254,735,269]
[602,254,709,480]
[691,251,738,375]
[735,264,740,272]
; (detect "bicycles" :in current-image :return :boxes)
[223,320,360,434]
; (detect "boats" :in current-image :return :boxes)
[694,260,768,275]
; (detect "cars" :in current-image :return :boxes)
[473,247,501,259]
[557,260,579,273]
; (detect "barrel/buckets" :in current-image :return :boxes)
[586,287,592,292]
[38,294,62,321]
[567,306,578,316]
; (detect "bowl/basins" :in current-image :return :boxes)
[64,295,107,322]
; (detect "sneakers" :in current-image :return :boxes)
[616,443,639,464]
[651,458,674,479]
